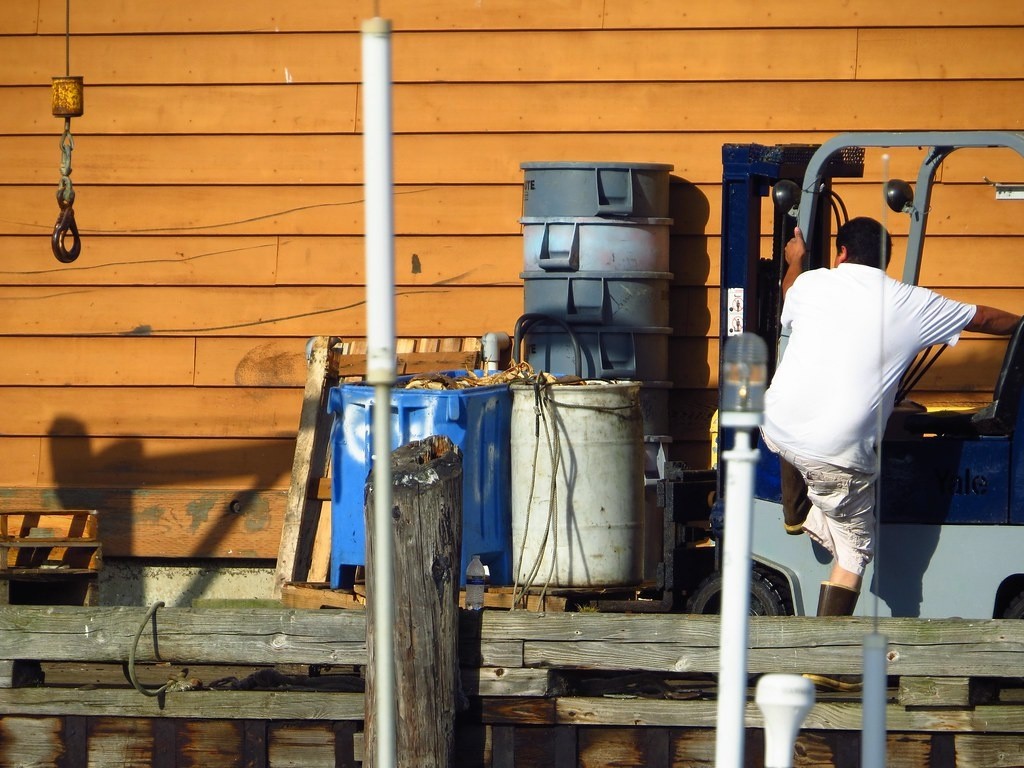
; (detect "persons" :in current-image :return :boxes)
[760,217,1024,692]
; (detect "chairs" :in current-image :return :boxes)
[879,314,1024,525]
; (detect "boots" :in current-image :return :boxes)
[779,455,813,535]
[802,581,863,692]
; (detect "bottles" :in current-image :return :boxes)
[465,556,484,608]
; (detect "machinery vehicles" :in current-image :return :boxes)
[586,129,1024,701]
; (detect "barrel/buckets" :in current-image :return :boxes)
[523,327,674,382]
[645,435,672,479]
[518,161,674,218]
[518,218,671,272]
[519,272,672,327]
[645,390,670,435]
[512,379,646,587]
[644,480,664,582]
